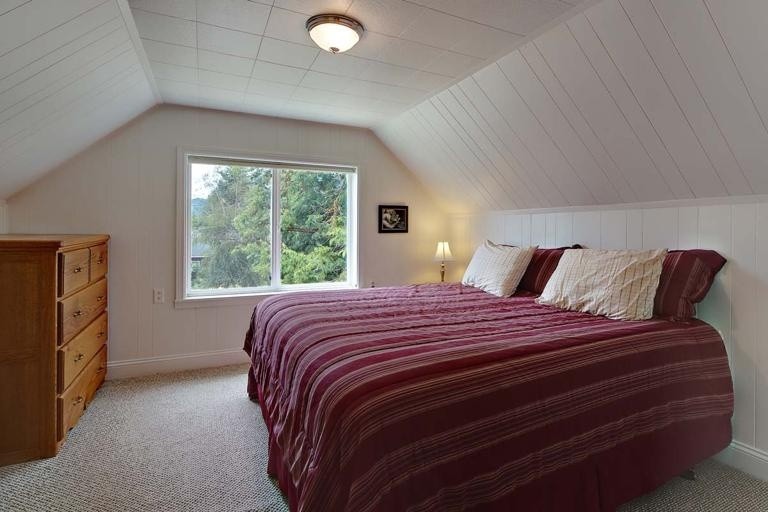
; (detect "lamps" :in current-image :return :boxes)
[434,240,455,281]
[304,13,366,57]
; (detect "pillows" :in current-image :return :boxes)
[460,239,536,300]
[518,244,580,297]
[535,247,665,322]
[654,250,728,326]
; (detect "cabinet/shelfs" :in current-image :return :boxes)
[3,233,110,465]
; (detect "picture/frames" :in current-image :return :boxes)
[377,204,408,233]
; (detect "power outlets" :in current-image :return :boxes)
[153,288,164,304]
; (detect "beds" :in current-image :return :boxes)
[243,283,735,511]
